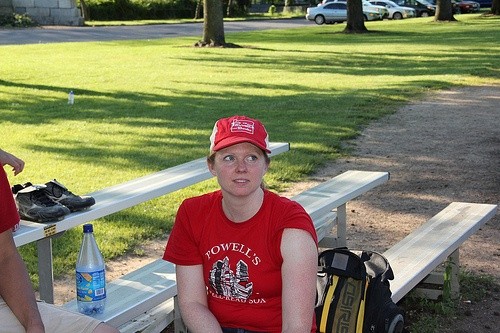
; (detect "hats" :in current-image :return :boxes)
[209,116,272,162]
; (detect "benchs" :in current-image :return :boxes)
[12,142,291,304]
[62,170,390,333]
[382,201,497,304]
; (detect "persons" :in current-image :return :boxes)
[0,148,25,176]
[162,116,319,333]
[0,161,120,333]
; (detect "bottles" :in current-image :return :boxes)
[75,224,106,316]
[68,90,74,105]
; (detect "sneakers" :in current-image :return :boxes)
[11,182,71,222]
[43,179,96,211]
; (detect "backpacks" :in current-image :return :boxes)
[309,247,406,333]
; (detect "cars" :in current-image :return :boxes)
[306,1,383,25]
[316,0,481,20]
